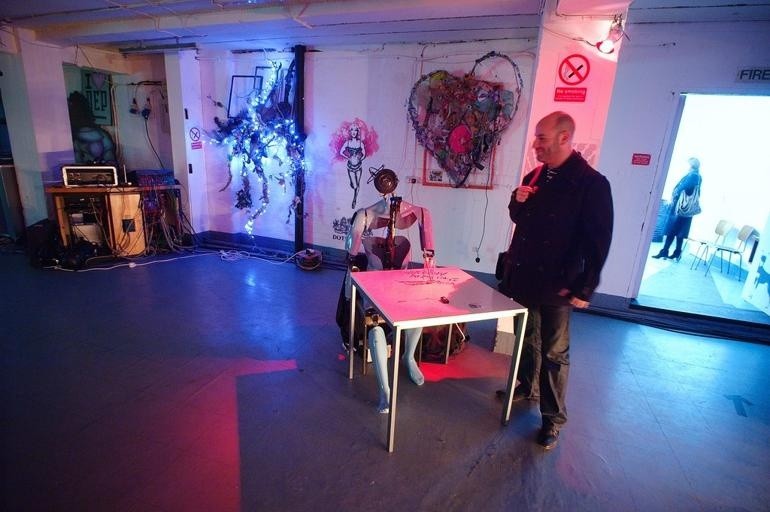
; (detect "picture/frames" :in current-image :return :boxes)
[80,69,114,126]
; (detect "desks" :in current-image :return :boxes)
[46,185,182,257]
[350,266,529,455]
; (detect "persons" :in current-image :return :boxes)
[493,111,614,447]
[340,124,366,209]
[651,157,703,261]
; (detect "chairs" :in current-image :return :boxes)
[356,299,422,376]
[676,218,755,282]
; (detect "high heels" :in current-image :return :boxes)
[651,249,681,261]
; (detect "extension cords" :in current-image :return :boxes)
[220,250,228,257]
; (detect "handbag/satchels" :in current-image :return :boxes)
[676,185,701,218]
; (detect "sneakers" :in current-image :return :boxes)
[496,387,541,402]
[537,424,560,449]
